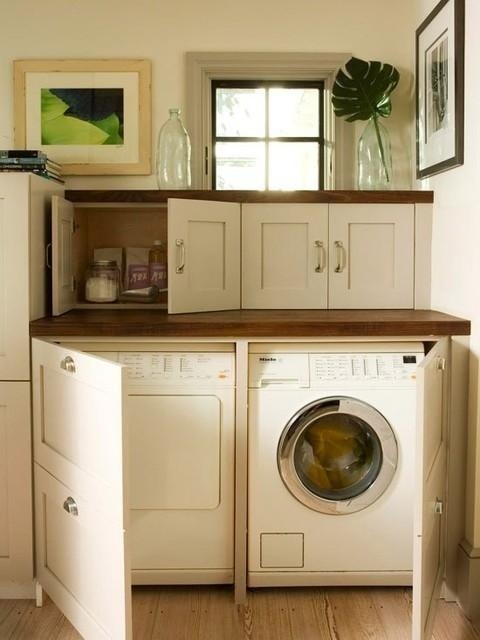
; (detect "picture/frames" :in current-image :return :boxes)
[413,0,465,180]
[11,57,153,178]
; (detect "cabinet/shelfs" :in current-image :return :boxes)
[29,336,239,640]
[49,193,240,314]
[242,202,417,311]
[242,333,455,640]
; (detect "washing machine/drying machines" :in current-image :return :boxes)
[248,343,425,589]
[60,343,235,589]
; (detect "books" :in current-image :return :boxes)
[0,150,65,185]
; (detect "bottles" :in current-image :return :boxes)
[84,260,120,303]
[149,239,168,303]
[355,113,393,190]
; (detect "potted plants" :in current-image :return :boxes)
[330,58,402,190]
